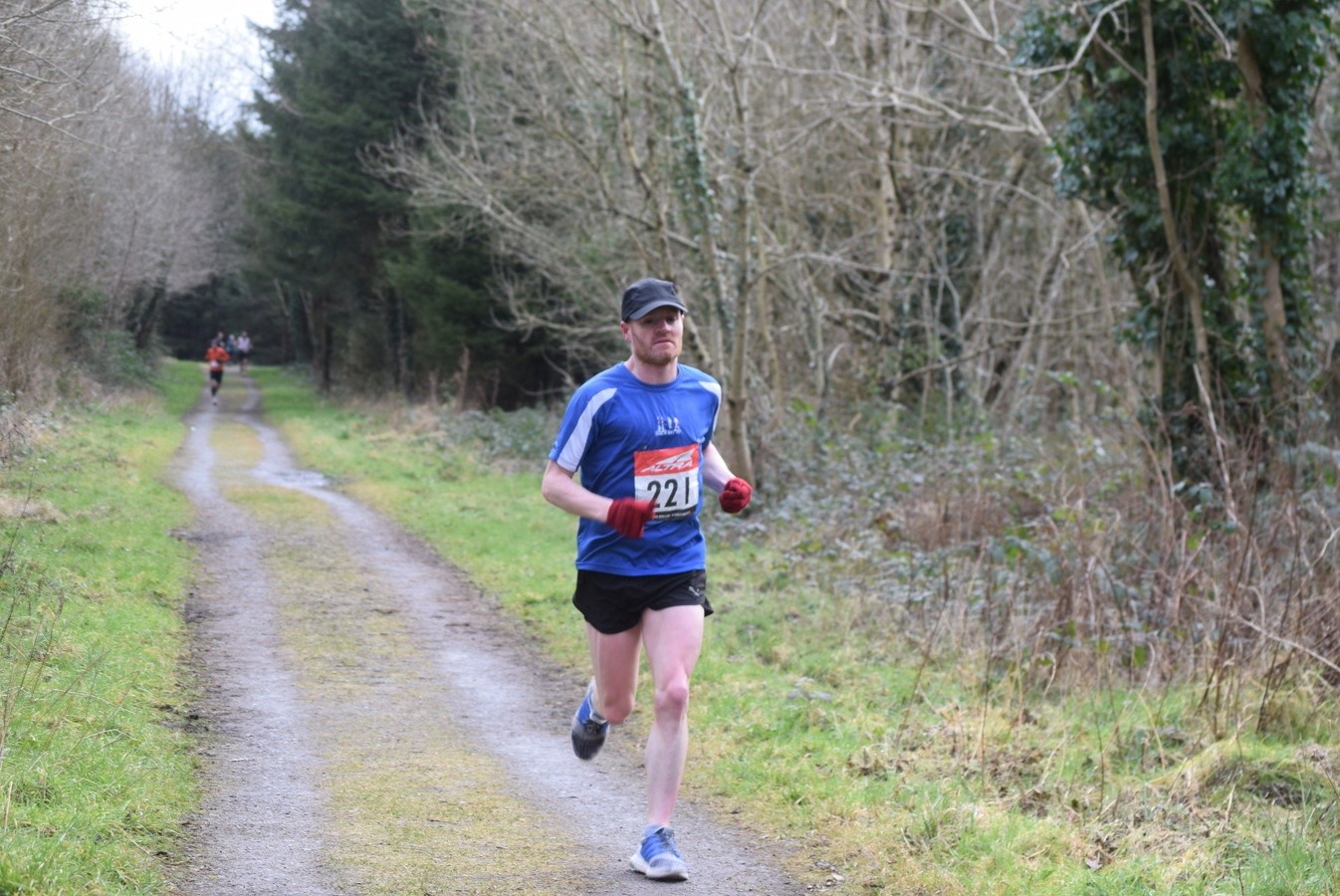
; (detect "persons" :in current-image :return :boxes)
[541,275,752,882]
[207,329,254,406]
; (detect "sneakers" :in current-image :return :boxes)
[630,824,687,881]
[570,677,611,762]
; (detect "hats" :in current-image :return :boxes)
[621,279,690,320]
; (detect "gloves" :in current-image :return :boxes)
[718,479,752,512]
[605,498,657,539]
